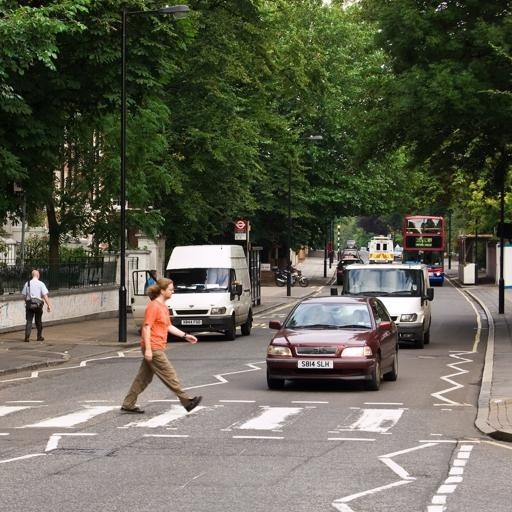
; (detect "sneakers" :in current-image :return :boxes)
[182,395,203,412]
[121,403,146,414]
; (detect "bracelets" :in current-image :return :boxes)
[183,334,189,339]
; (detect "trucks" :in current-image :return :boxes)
[367,235,395,264]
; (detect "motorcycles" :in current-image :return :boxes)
[271,266,308,287]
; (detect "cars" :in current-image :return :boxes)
[266,296,400,391]
[337,239,364,284]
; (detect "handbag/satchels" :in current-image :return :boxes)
[28,298,44,310]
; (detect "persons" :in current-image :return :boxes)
[121,278,204,414]
[144,270,158,295]
[21,270,51,342]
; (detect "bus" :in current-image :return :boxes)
[403,215,445,285]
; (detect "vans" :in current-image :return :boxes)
[330,264,434,349]
[130,244,253,341]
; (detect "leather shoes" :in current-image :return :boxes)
[24,337,30,342]
[37,336,45,341]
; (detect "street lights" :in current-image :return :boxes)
[287,135,323,296]
[118,4,190,343]
